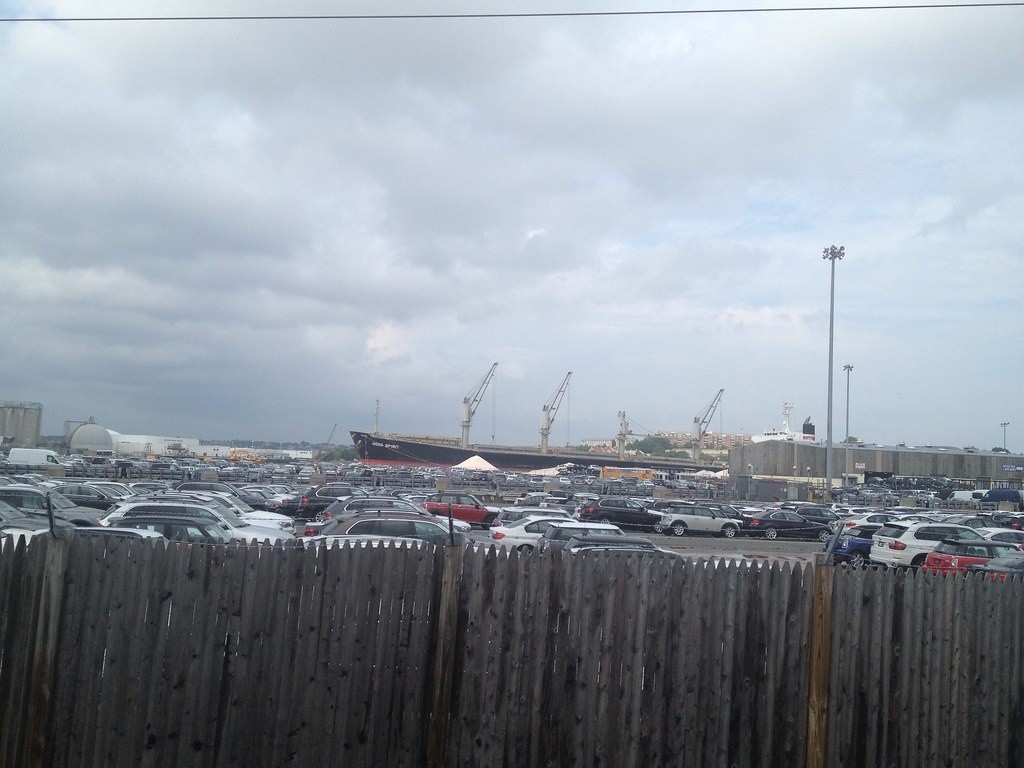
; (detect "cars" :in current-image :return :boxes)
[1,446,1024,584]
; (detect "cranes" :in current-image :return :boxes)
[540,371,574,453]
[692,386,725,464]
[462,362,500,449]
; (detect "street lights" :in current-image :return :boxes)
[1000,420,1010,453]
[821,242,846,502]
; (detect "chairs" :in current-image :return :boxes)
[176,530,185,541]
[968,548,976,554]
[977,549,985,554]
